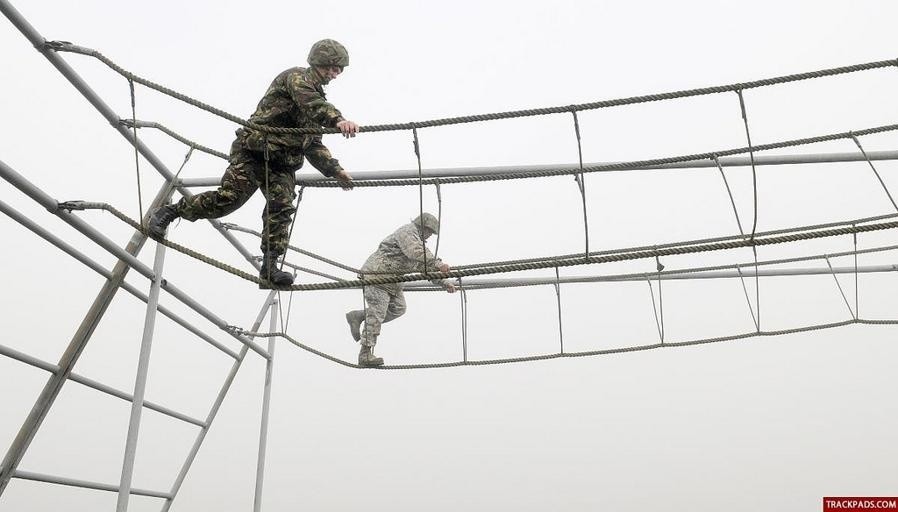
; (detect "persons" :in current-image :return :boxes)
[148,38,361,286]
[346,213,456,367]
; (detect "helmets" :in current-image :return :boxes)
[413,213,440,235]
[307,38,349,66]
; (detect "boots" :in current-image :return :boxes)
[145,204,179,238]
[259,256,293,284]
[346,309,365,341]
[358,345,384,365]
